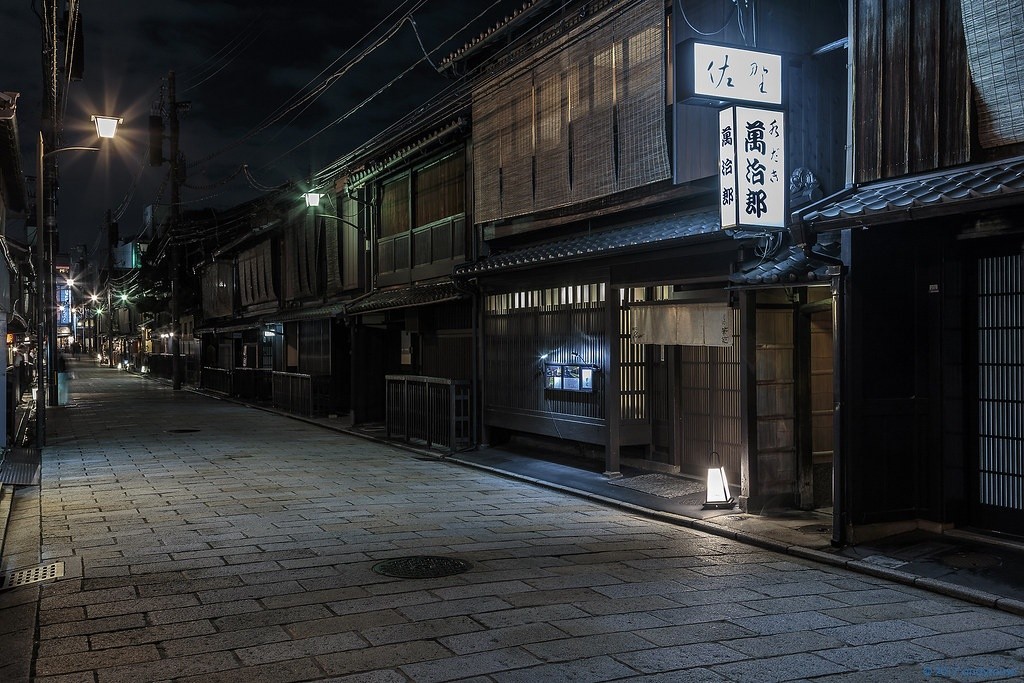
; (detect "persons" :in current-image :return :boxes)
[70,340,81,361]
[14,344,36,406]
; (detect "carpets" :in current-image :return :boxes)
[607,473,707,499]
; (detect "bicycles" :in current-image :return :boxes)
[70,342,80,361]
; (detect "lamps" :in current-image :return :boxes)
[299,189,365,236]
[571,352,597,373]
[701,452,735,509]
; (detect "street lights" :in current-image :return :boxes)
[301,191,365,428]
[35,114,123,453]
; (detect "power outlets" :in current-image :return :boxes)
[794,293,799,302]
